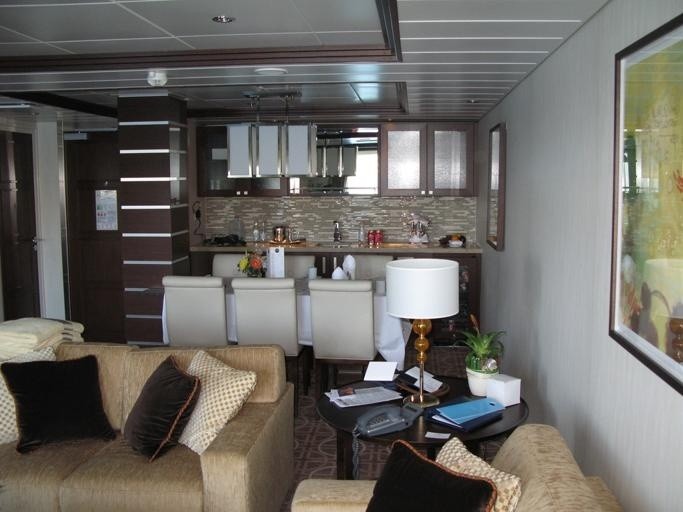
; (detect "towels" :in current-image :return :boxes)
[0,316,85,351]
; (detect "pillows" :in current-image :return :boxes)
[433,437,518,512]
[120,350,201,465]
[180,347,258,457]
[357,436,496,512]
[0,352,116,454]
[0,342,58,447]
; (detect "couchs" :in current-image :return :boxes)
[0,339,299,512]
[287,419,625,512]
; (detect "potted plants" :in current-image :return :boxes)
[457,326,508,398]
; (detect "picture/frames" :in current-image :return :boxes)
[608,14,682,397]
[484,121,508,250]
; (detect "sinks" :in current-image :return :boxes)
[337,243,363,248]
[317,244,338,249]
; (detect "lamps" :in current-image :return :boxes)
[382,256,462,415]
[223,85,317,182]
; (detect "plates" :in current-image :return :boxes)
[269,239,286,244]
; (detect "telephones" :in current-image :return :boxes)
[355,402,424,437]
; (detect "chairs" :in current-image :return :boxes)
[158,253,414,401]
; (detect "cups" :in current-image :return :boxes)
[304,231,318,247]
[308,267,316,280]
[287,229,299,244]
[375,280,385,296]
[272,227,284,242]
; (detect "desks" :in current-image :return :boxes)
[312,377,531,482]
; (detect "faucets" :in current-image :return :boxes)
[334,222,342,241]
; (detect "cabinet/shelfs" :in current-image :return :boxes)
[196,125,287,198]
[380,123,474,197]
[395,253,480,345]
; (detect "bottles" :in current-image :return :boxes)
[368,230,384,245]
[229,215,241,240]
[251,217,266,241]
